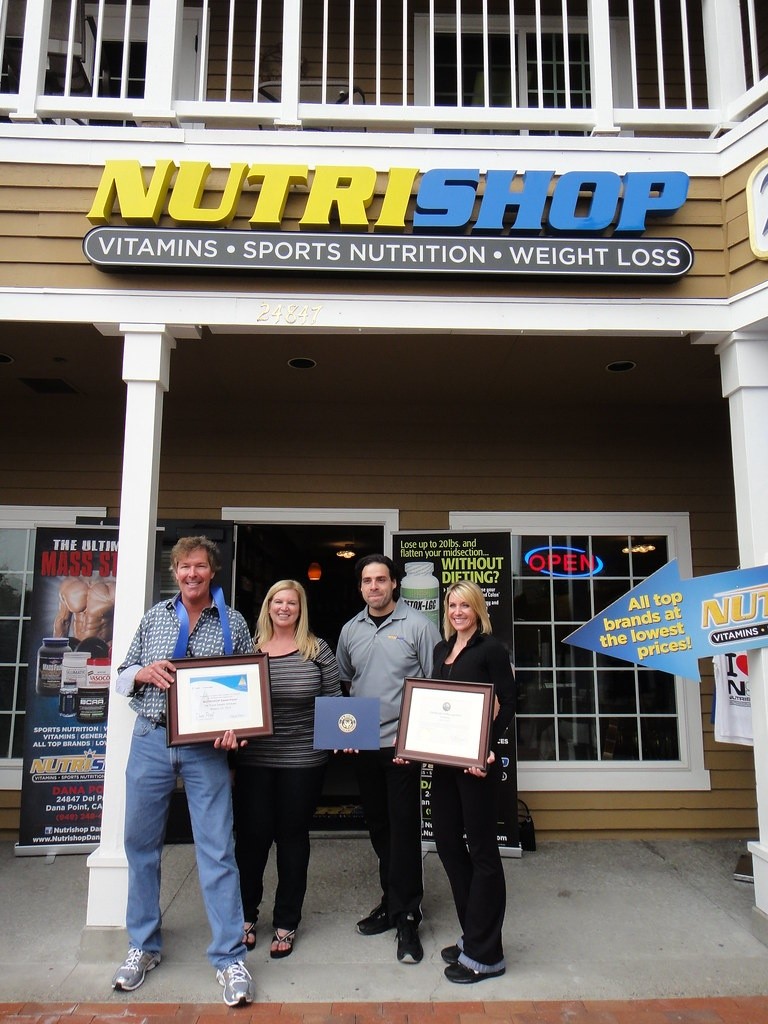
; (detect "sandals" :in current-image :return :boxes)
[241,922,256,951]
[270,927,296,958]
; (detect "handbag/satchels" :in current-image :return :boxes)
[517,798,536,852]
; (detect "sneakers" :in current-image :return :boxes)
[215,960,255,1006]
[356,896,398,935]
[394,912,424,963]
[110,945,161,991]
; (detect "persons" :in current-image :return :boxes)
[52,570,115,650]
[113,535,256,1007]
[234,579,341,959]
[335,555,445,964]
[393,581,521,985]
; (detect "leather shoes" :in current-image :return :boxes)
[441,945,463,965]
[444,961,506,983]
